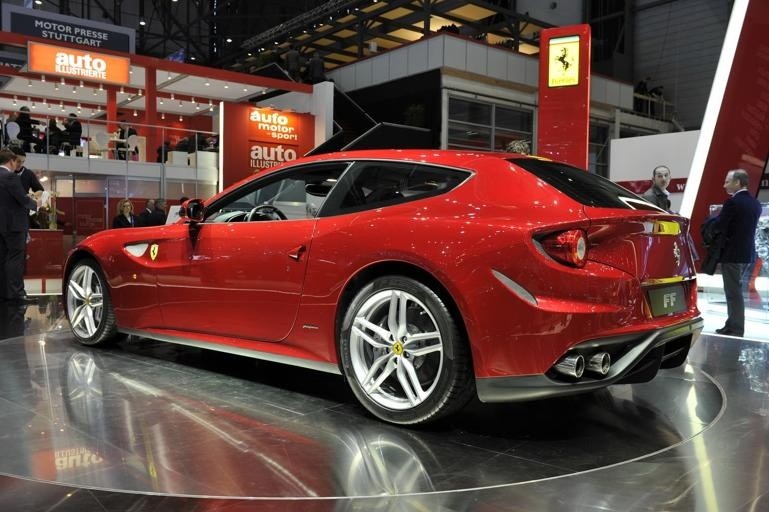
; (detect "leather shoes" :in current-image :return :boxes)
[6,297,39,304]
[716,325,744,337]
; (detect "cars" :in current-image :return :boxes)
[59,333,698,512]
[62,146,706,433]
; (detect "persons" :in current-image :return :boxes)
[4,106,82,157]
[118,120,138,160]
[646,86,664,115]
[271,44,325,85]
[156,136,192,163]
[0,149,40,304]
[112,198,168,228]
[634,77,651,113]
[642,166,671,212]
[11,147,44,198]
[711,170,762,338]
